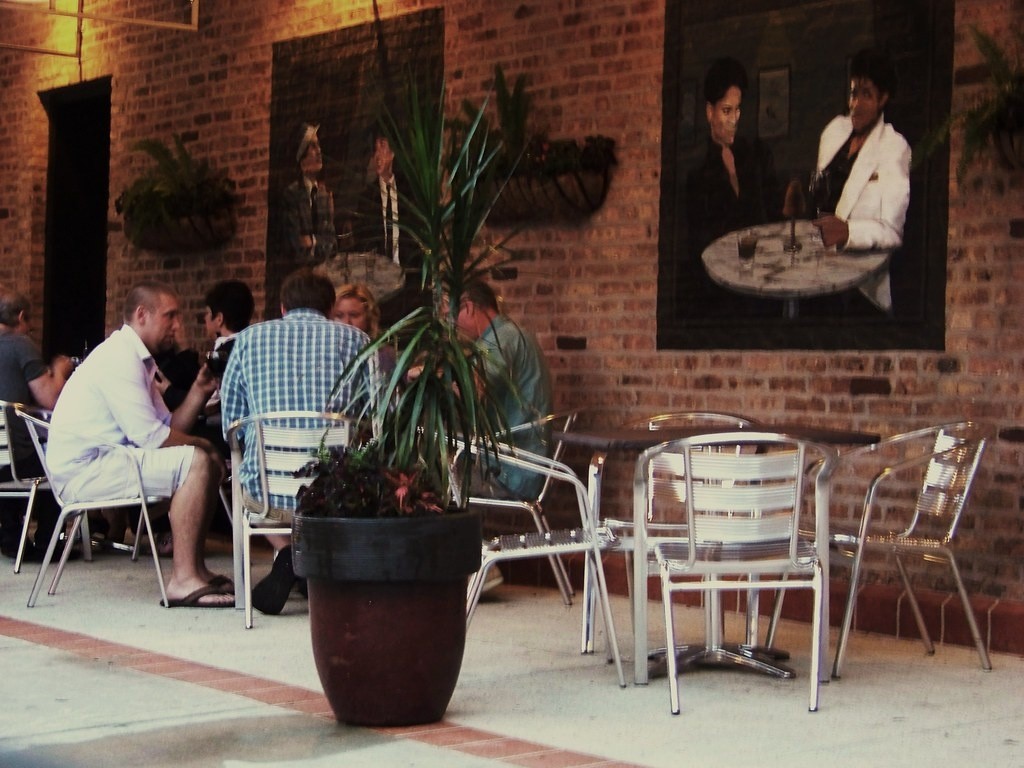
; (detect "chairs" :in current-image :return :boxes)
[581,408,767,655]
[634,431,840,714]
[228,411,376,629]
[448,407,582,607]
[16,406,168,608]
[0,401,93,574]
[766,419,994,681]
[416,423,625,690]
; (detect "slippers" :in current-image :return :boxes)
[161,583,236,609]
[207,574,235,593]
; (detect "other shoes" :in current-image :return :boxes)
[0,524,38,563]
[250,545,297,614]
[296,578,309,599]
[34,533,85,561]
[463,566,504,594]
[156,534,174,559]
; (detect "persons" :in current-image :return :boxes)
[407,278,553,592]
[220,269,383,613]
[802,51,913,326]
[45,279,234,608]
[328,284,395,377]
[352,114,430,305]
[203,279,254,377]
[281,122,337,267]
[686,59,805,321]
[0,291,74,564]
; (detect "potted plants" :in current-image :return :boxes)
[439,62,618,223]
[289,55,559,726]
[911,20,1023,191]
[115,133,237,257]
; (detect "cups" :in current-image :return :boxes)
[736,232,759,266]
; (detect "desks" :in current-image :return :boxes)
[555,425,881,681]
[311,251,406,305]
[702,219,890,318]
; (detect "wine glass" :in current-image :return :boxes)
[205,350,228,399]
[809,169,830,237]
